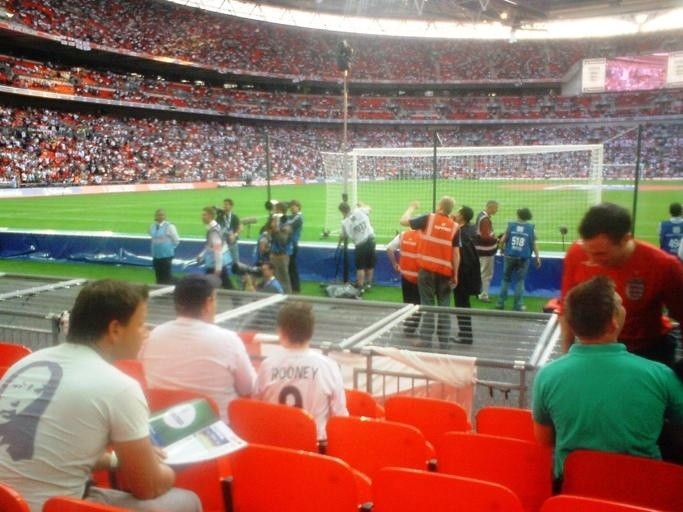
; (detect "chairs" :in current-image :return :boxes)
[0,340,681,512]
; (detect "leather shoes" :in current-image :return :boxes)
[404,331,473,348]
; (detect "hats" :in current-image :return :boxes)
[174,272,222,298]
[517,208,531,220]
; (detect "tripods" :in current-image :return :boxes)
[320,237,348,287]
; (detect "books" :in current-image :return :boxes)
[103,395,250,470]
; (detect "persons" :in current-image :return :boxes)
[554,202,682,372]
[148,209,178,282]
[530,272,683,494]
[242,199,303,296]
[200,198,243,309]
[136,271,258,426]
[0,276,202,512]
[0,1,683,188]
[338,201,377,292]
[475,200,541,310]
[659,203,680,256]
[387,196,482,351]
[253,298,350,456]
